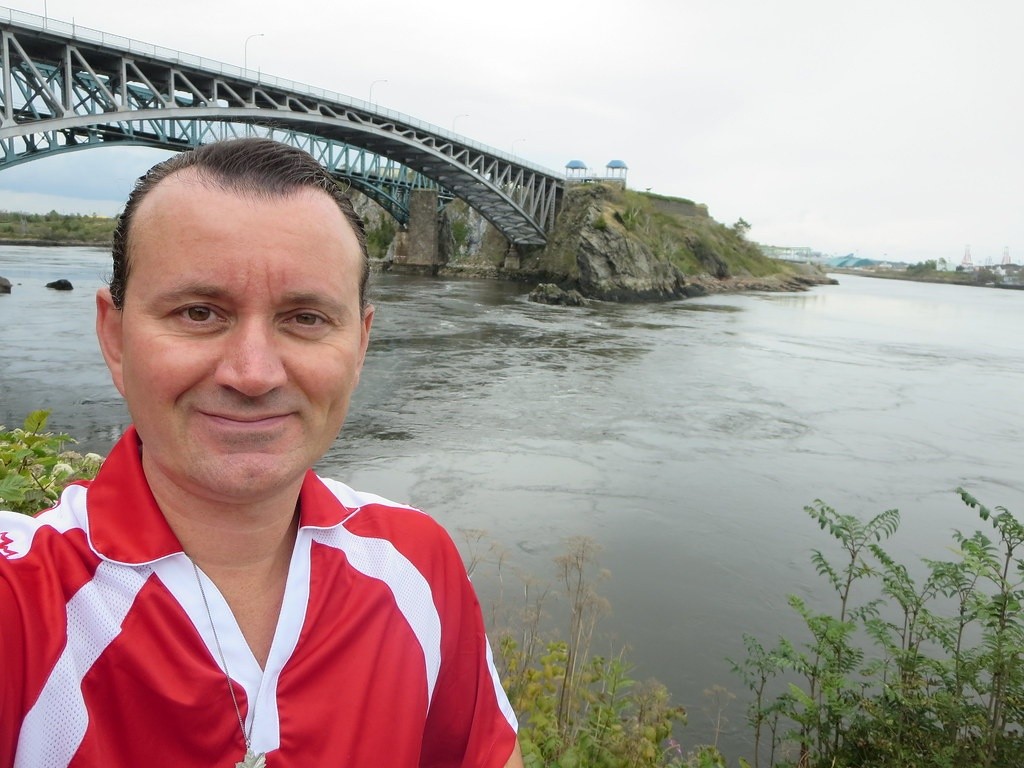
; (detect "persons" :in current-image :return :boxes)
[1,137,525,768]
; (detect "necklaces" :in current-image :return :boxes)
[188,553,267,768]
